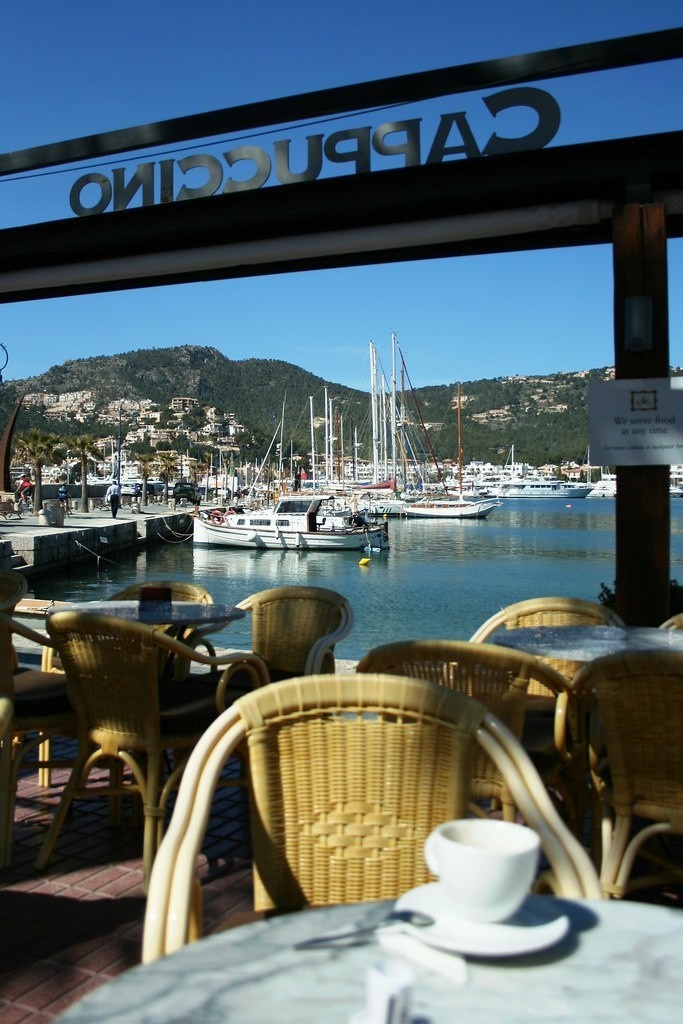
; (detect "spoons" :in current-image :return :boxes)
[294,908,434,951]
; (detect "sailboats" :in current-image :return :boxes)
[189,330,617,551]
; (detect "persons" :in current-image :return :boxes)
[58,483,69,507]
[14,474,34,506]
[105,481,121,518]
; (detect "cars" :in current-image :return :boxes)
[120,482,141,497]
[162,486,175,497]
[139,483,154,496]
[213,487,231,499]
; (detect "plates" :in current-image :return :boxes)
[394,882,570,956]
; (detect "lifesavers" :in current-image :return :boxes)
[224,512,236,517]
[210,510,224,525]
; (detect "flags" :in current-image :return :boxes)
[301,468,307,481]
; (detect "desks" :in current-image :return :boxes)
[51,897,683,1024]
[50,601,246,777]
[496,624,683,848]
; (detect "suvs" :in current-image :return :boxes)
[171,482,202,506]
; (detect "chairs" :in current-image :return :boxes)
[0,584,683,969]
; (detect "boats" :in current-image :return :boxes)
[670,481,683,499]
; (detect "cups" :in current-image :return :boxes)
[424,818,541,922]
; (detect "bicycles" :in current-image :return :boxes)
[15,490,34,507]
[62,497,73,518]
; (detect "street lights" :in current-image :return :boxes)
[116,397,141,491]
